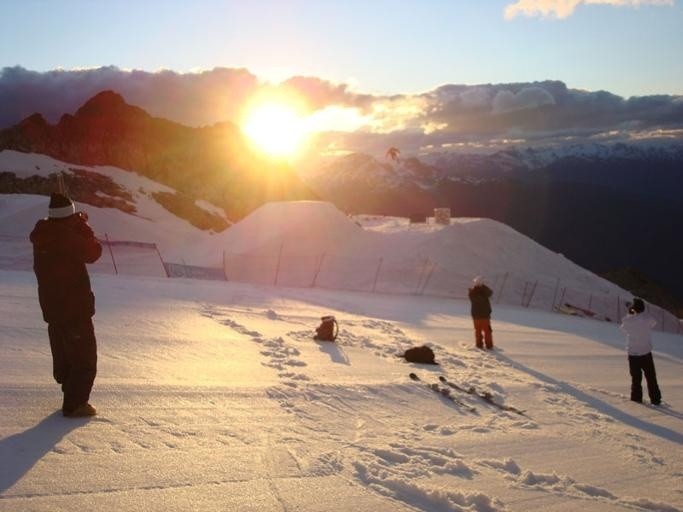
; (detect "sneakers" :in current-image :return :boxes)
[66,400,95,416]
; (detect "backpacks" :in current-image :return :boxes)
[315,316,338,341]
[405,346,438,365]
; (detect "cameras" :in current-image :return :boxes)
[76,211,88,221]
[624,301,635,314]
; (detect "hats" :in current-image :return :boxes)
[473,277,483,286]
[632,298,646,313]
[47,192,74,219]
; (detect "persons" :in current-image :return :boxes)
[619,297,663,406]
[28,189,102,418]
[468,276,494,349]
